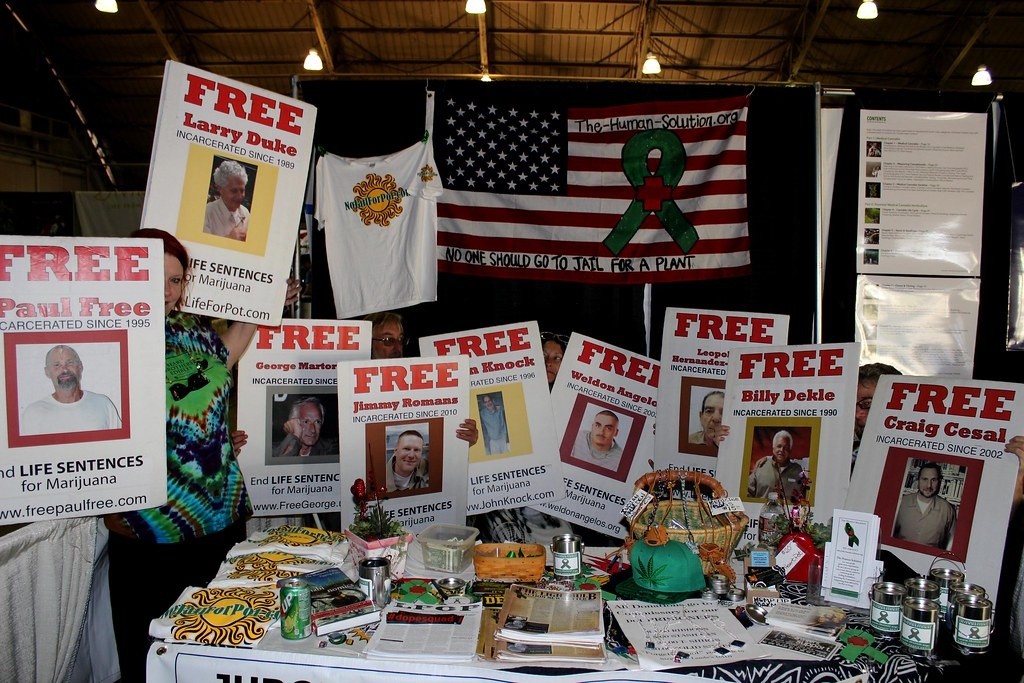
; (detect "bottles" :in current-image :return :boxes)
[759,492,787,557]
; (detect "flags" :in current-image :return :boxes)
[425,92,752,285]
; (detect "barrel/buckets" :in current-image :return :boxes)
[359,555,392,609]
[905,577,939,640]
[952,595,995,653]
[901,596,941,657]
[550,534,584,581]
[927,551,967,622]
[868,582,907,638]
[948,582,989,638]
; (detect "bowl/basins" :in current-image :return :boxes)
[417,523,480,574]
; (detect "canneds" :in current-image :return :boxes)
[279,578,312,640]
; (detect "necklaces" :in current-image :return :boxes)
[587,435,609,460]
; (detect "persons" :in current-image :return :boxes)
[271,396,340,458]
[480,395,509,454]
[715,363,1024,505]
[893,462,956,551]
[387,430,429,493]
[487,331,574,546]
[204,160,250,243]
[101,226,303,683]
[19,345,124,435]
[689,391,725,445]
[572,410,623,473]
[363,312,478,448]
[747,430,809,502]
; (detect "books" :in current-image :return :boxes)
[764,603,850,642]
[477,583,608,665]
[275,566,382,637]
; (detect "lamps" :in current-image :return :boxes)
[971,63,993,87]
[856,0,877,20]
[642,47,661,76]
[302,49,324,71]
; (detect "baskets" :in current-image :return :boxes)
[625,470,749,577]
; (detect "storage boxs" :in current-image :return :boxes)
[472,539,546,580]
[417,522,480,572]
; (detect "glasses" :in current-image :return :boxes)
[168,372,210,401]
[540,331,570,346]
[856,397,873,410]
[372,335,410,346]
